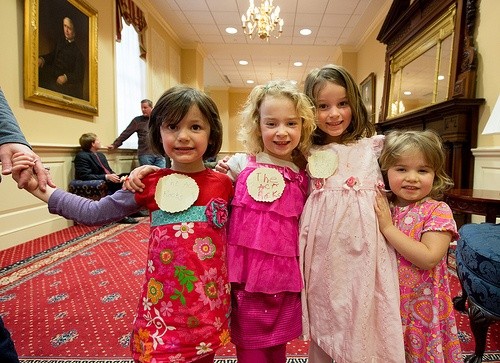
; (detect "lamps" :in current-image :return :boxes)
[241,1,284,43]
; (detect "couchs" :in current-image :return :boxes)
[69,180,107,200]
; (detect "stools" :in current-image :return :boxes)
[453,223,500,363]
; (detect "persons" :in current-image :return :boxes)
[120,81,317,363]
[38,16,88,96]
[0,83,56,363]
[374,131,464,363]
[11,84,236,363]
[215,64,405,363]
[75,132,151,224]
[106,99,167,169]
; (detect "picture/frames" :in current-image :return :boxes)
[23,0,99,117]
[358,72,376,125]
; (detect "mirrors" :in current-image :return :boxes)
[385,2,456,121]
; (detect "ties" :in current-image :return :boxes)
[96,155,111,174]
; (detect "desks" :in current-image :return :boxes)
[446,188,500,223]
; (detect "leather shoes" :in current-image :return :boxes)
[129,211,149,218]
[116,216,139,224]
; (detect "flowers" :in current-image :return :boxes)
[205,197,228,228]
[343,176,362,191]
[376,182,385,191]
[311,177,325,194]
